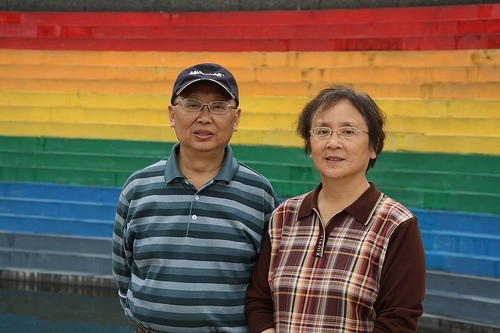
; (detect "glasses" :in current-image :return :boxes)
[309,126,371,138]
[173,98,236,115]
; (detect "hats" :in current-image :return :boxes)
[171,62,239,105]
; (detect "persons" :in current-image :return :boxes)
[108,61,280,333]
[239,80,428,333]
